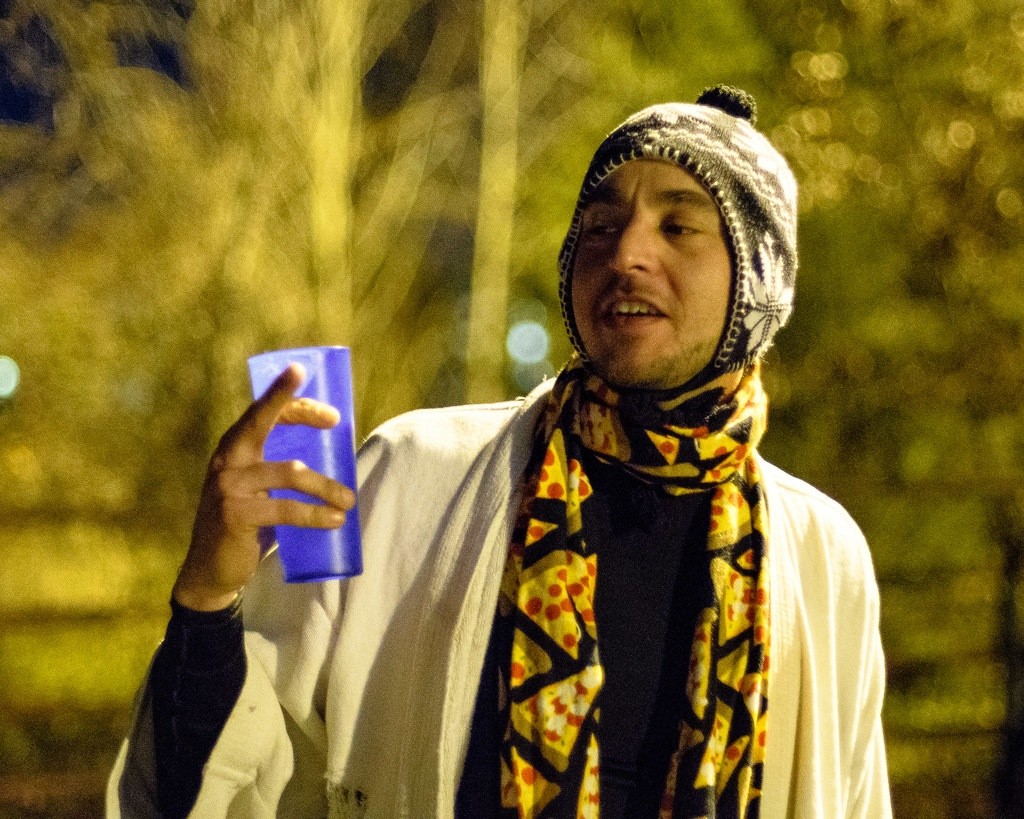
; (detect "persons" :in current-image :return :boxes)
[106,83,894,819]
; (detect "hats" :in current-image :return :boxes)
[556,84,799,400]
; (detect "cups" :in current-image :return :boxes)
[246,345,365,583]
